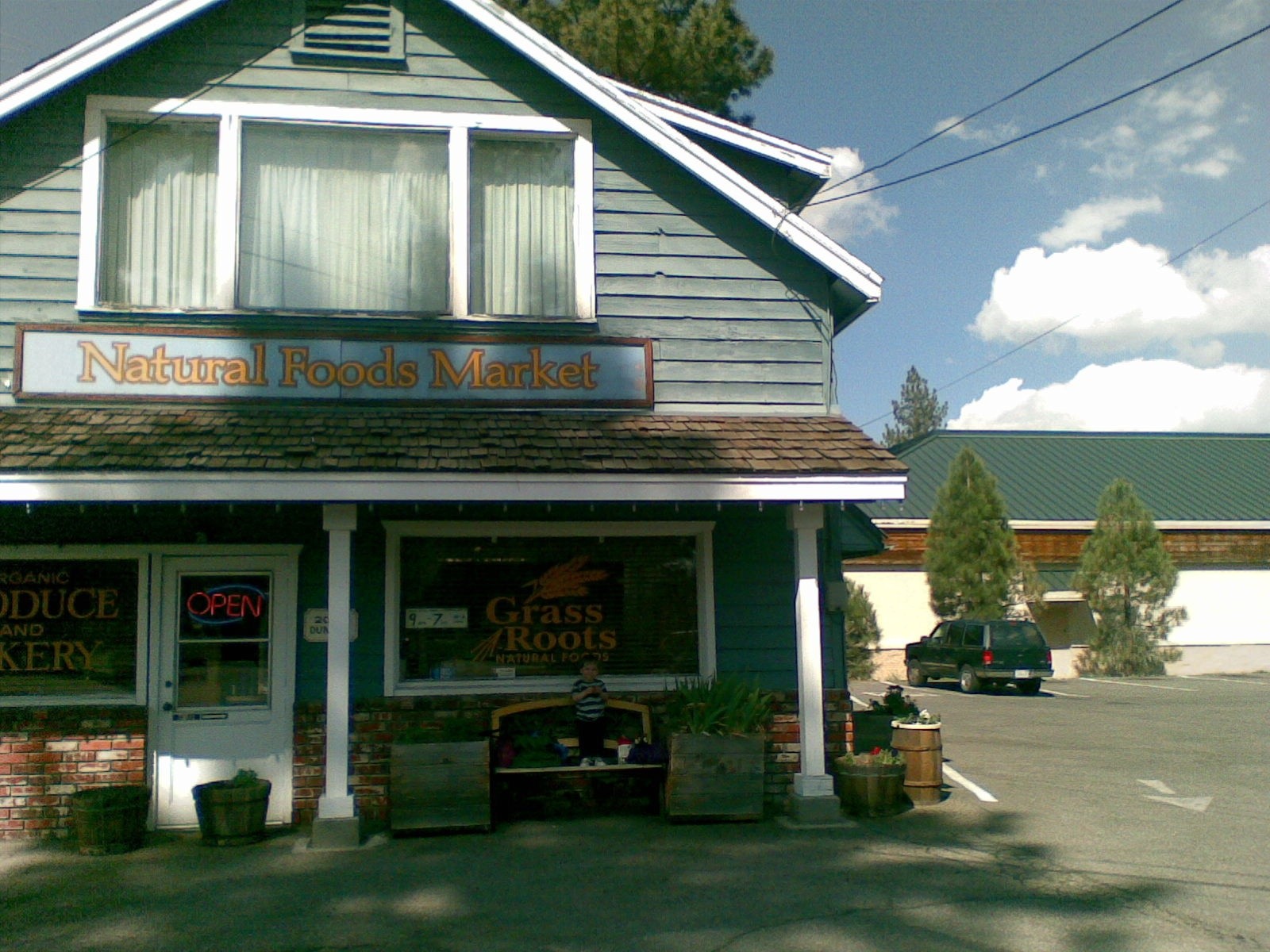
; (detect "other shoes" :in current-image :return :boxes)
[580,758,590,766]
[595,760,607,767]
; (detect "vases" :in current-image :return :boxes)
[836,754,905,817]
[851,711,898,754]
[72,785,153,854]
[891,720,943,805]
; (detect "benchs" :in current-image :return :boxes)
[489,697,664,819]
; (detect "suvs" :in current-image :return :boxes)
[905,617,1055,696]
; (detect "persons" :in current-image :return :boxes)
[571,653,609,767]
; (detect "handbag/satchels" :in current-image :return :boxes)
[626,743,662,764]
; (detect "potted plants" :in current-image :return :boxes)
[390,715,491,834]
[192,768,271,846]
[663,669,772,821]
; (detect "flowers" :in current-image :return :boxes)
[894,708,941,725]
[845,746,902,766]
[868,685,918,714]
